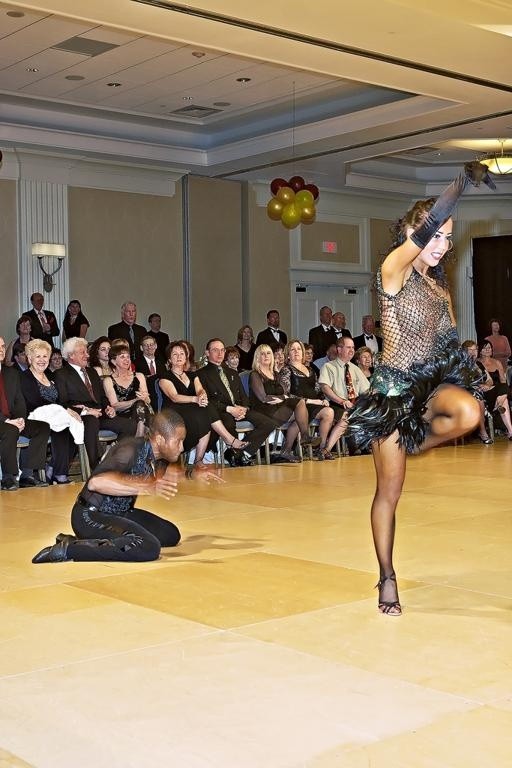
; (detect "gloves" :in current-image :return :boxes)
[409,159,497,249]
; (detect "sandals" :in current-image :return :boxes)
[227,438,250,450]
[377,571,400,616]
[193,459,208,471]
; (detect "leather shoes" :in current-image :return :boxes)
[224,447,252,466]
[32,533,76,563]
[479,433,493,445]
[1,475,72,490]
[281,435,335,462]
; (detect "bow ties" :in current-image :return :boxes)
[366,335,373,340]
[335,331,342,335]
[273,328,280,333]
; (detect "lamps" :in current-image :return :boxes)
[475,139,512,175]
[33,243,66,293]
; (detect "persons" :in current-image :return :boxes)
[0,293,170,490]
[341,161,499,617]
[308,306,382,358]
[256,310,288,347]
[31,406,230,566]
[461,320,512,445]
[159,326,378,470]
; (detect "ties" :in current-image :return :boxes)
[345,363,356,404]
[38,310,46,327]
[129,324,135,344]
[81,368,97,403]
[217,365,235,406]
[150,359,155,375]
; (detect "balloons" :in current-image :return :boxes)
[266,175,321,230]
